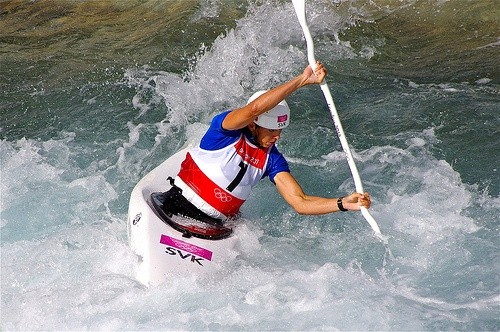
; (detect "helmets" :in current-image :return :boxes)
[247,90,290,130]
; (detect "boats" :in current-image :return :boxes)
[125,145,239,289]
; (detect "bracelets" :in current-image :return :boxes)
[337,197,348,212]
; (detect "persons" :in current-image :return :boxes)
[162,60,371,240]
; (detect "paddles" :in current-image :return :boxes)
[291,0,387,237]
